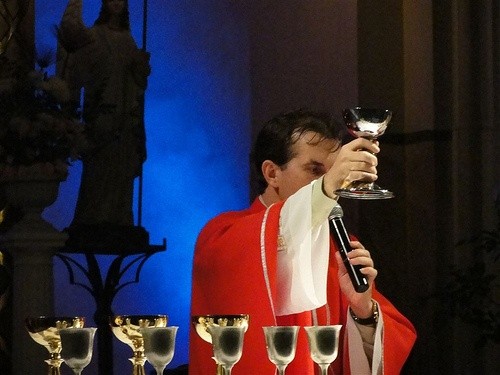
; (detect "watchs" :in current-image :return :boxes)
[349,300,382,324]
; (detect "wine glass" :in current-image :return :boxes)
[305,325,343,374]
[262,326,299,375]
[23,314,85,375]
[143,326,178,375]
[108,313,169,375]
[333,105,395,200]
[190,313,250,375]
[58,329,96,375]
[210,326,246,375]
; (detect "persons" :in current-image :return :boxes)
[189,107,418,375]
[60,0,152,227]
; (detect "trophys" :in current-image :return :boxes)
[109,315,169,375]
[193,314,249,375]
[209,327,245,375]
[57,328,98,375]
[304,325,343,375]
[21,316,86,375]
[261,326,301,375]
[334,106,397,199]
[141,327,179,375]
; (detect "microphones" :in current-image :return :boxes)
[328,202,369,293]
